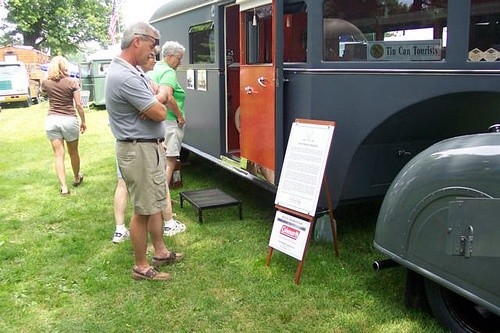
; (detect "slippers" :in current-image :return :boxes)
[72,176,83,186]
[152,251,184,267]
[60,188,70,197]
[132,268,170,282]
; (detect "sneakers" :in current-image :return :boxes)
[112,230,131,243]
[162,220,186,237]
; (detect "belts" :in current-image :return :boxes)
[119,138,164,144]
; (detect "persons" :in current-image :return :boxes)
[113,47,186,244]
[150,40,190,187]
[39,56,87,196]
[105,21,183,281]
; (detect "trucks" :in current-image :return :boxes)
[145,0,500,333]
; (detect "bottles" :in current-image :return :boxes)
[314,213,338,242]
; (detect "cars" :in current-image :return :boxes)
[0,60,42,109]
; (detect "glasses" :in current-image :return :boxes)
[135,33,160,47]
